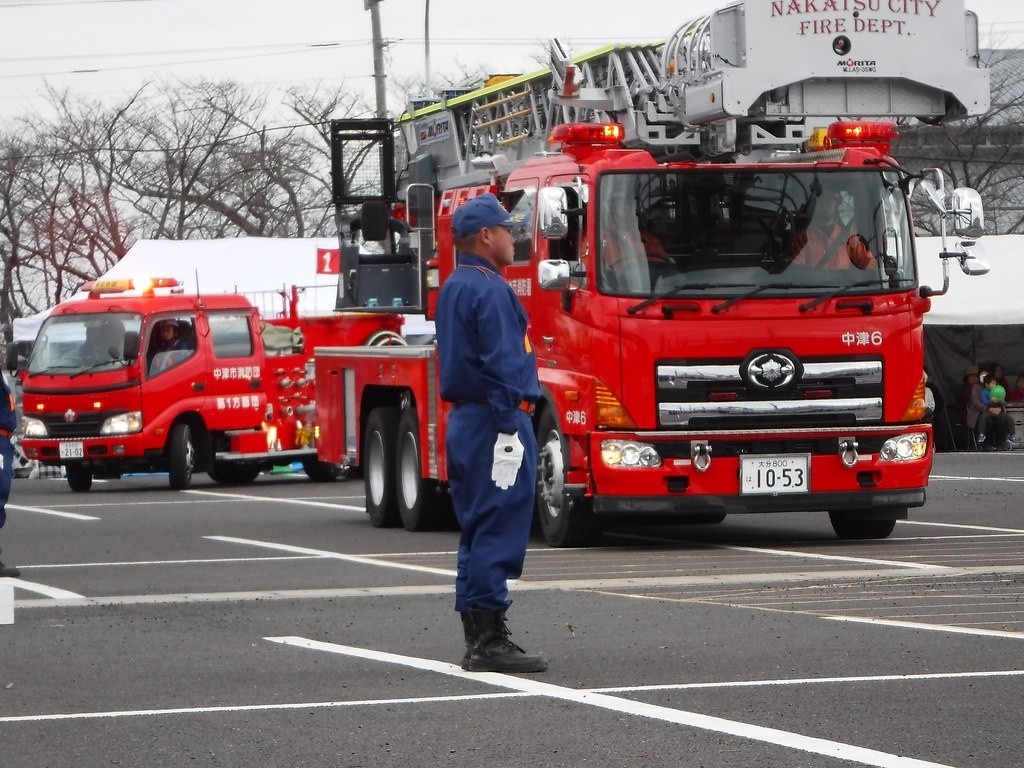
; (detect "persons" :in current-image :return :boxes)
[0,350,21,577]
[924,370,935,453]
[147,319,186,375]
[435,192,550,673]
[579,189,676,272]
[75,320,112,367]
[791,185,877,271]
[959,363,1024,451]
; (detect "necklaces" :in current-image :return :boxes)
[458,264,509,284]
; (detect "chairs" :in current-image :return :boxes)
[944,402,1007,451]
[174,320,194,344]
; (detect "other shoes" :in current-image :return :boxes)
[983,442,994,452]
[0,561,19,577]
[997,442,1007,450]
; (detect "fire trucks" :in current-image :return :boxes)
[5,268,412,492]
[314,1,988,544]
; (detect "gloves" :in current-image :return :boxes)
[491,430,525,490]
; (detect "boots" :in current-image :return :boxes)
[459,610,474,670]
[468,605,546,672]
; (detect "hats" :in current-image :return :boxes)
[963,366,979,381]
[451,192,529,237]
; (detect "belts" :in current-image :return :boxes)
[457,397,536,417]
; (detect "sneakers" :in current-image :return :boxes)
[1007,436,1018,445]
[978,434,986,443]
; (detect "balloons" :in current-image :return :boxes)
[990,385,1006,403]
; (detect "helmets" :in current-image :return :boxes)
[809,177,841,201]
[84,316,109,327]
[604,181,639,205]
[159,318,178,327]
